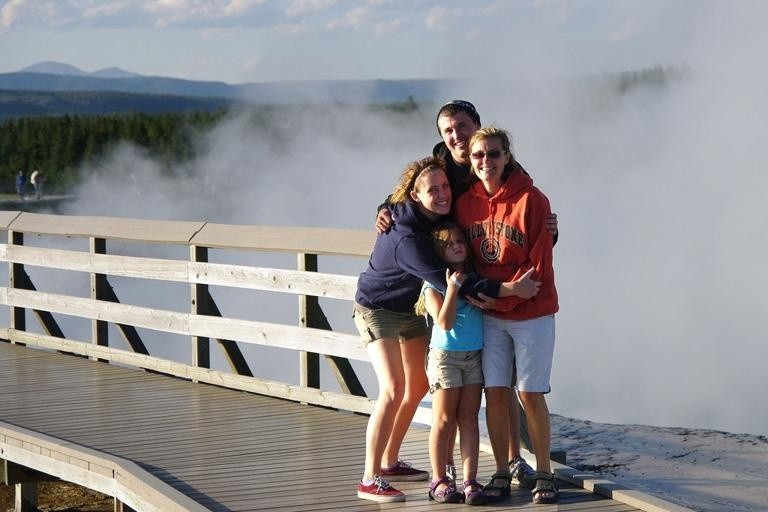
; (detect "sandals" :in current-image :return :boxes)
[428,457,559,505]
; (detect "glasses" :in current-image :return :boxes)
[471,151,504,158]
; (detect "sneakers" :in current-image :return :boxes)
[357,475,405,502]
[382,461,429,482]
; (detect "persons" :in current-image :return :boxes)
[351,157,540,504]
[15,169,27,200]
[413,221,485,504]
[375,99,559,488]
[30,168,43,199]
[452,128,558,504]
